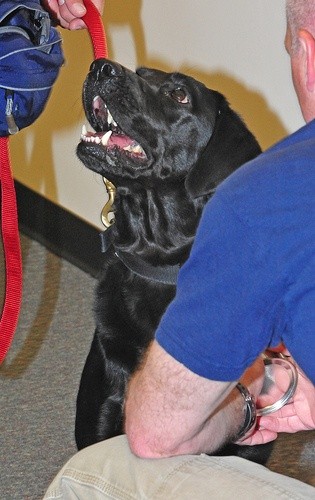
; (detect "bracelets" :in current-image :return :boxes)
[254,357,297,417]
[234,382,256,437]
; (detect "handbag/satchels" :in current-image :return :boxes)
[0,0,65,138]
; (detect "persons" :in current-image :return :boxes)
[39,2,315,500]
[0,0,104,136]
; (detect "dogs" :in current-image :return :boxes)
[74,58,274,468]
